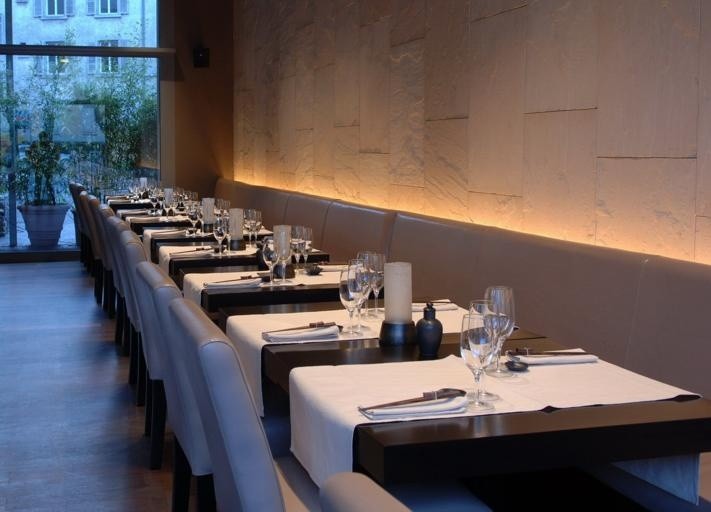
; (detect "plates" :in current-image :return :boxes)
[506,360,530,372]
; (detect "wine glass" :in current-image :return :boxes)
[340,252,388,337]
[463,284,518,411]
[125,176,325,288]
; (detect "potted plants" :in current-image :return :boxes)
[19,130,71,246]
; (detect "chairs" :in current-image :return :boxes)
[68,181,326,511]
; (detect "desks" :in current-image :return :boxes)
[106,190,710,448]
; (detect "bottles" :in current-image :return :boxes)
[414,302,446,359]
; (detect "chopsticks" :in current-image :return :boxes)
[361,385,468,411]
[412,298,459,306]
[265,320,337,334]
[508,346,597,356]
